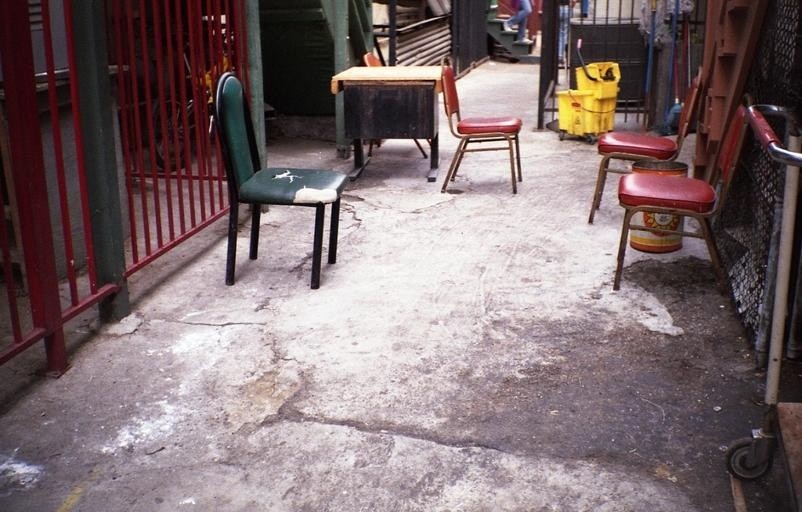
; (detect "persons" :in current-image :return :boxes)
[503,0,534,45]
[558,0,589,69]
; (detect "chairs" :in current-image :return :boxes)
[613,93,748,296]
[363,52,433,158]
[216,70,348,290]
[440,54,523,194]
[588,62,703,225]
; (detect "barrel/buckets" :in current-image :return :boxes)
[630,159,689,253]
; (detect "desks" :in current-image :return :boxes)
[331,63,444,185]
